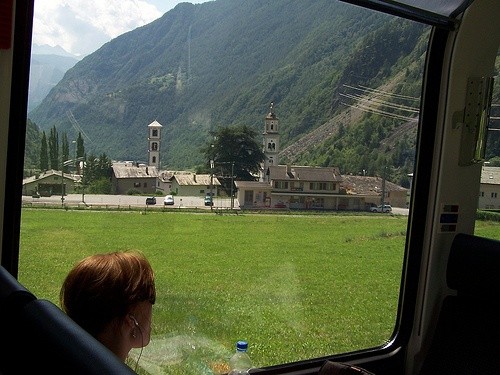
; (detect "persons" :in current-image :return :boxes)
[60,249,156,364]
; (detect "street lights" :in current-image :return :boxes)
[61,155,65,206]
[72,140,76,176]
[211,160,214,210]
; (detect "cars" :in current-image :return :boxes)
[372,204,392,213]
[204,196,214,206]
[146,197,156,204]
[42,191,50,197]
[32,192,40,198]
[164,194,174,205]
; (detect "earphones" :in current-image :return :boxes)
[129,314,138,326]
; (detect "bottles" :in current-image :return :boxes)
[227,341,252,375]
